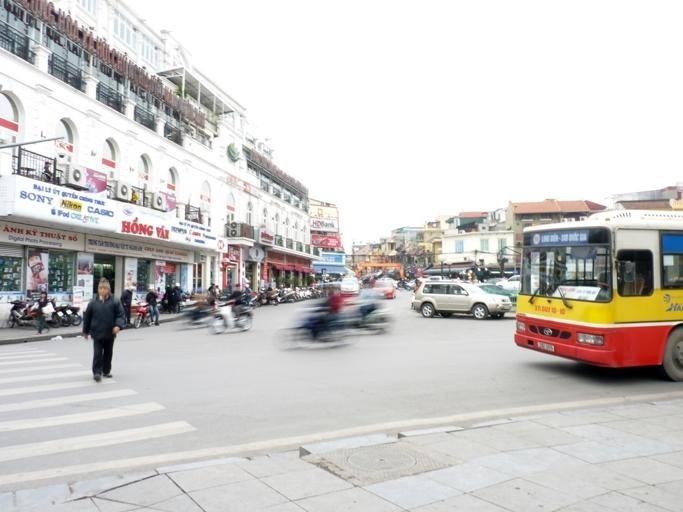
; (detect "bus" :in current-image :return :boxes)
[513,209,683,381]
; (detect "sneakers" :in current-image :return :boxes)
[103,373,112,377]
[94,373,101,382]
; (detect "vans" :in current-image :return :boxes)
[340,277,360,296]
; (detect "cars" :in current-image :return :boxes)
[412,274,520,320]
[372,280,396,298]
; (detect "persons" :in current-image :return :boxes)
[229,284,245,322]
[161,283,185,313]
[29,291,55,332]
[208,283,221,296]
[83,278,126,382]
[121,285,135,323]
[146,284,161,327]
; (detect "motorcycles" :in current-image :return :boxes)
[250,286,324,307]
[134,302,152,328]
[7,298,82,328]
[211,304,253,334]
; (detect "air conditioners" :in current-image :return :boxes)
[63,164,166,211]
[228,222,240,236]
